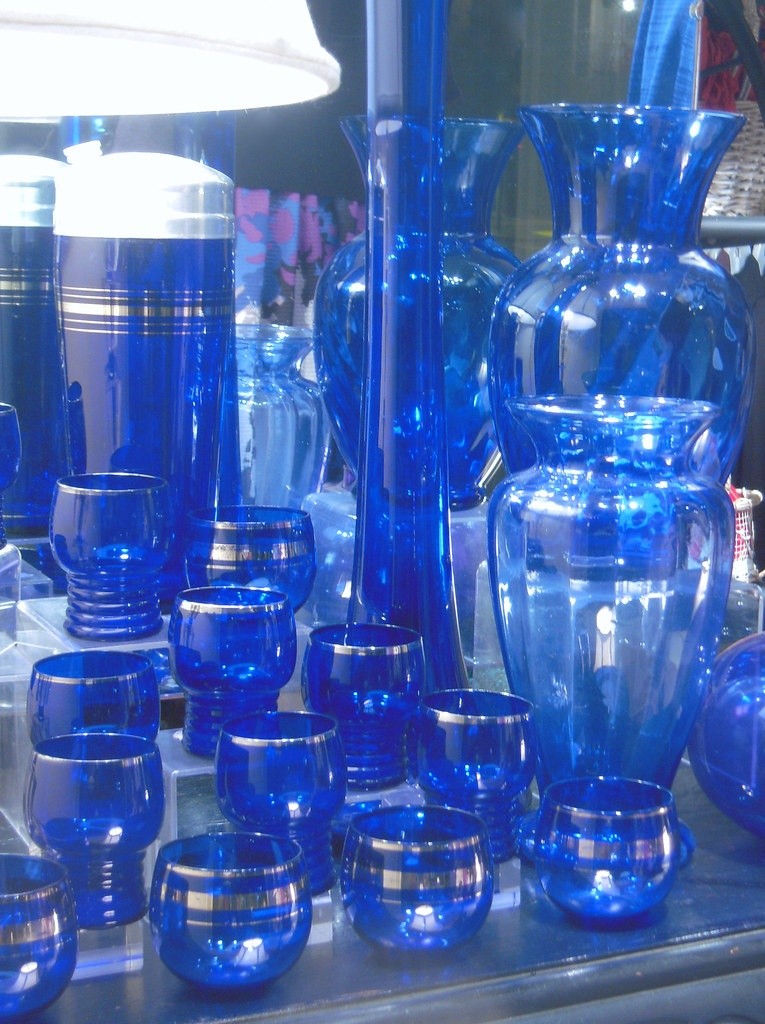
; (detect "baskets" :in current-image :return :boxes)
[702,100,765,219]
[687,488,762,585]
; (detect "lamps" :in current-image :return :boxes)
[0,0,344,206]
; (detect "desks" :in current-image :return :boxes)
[2,589,764,1024]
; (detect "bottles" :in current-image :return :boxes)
[231,104,763,869]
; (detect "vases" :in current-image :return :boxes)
[234,321,327,509]
[493,103,752,484]
[485,390,739,786]
[312,112,522,509]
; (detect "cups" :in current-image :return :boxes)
[0,469,678,1024]
